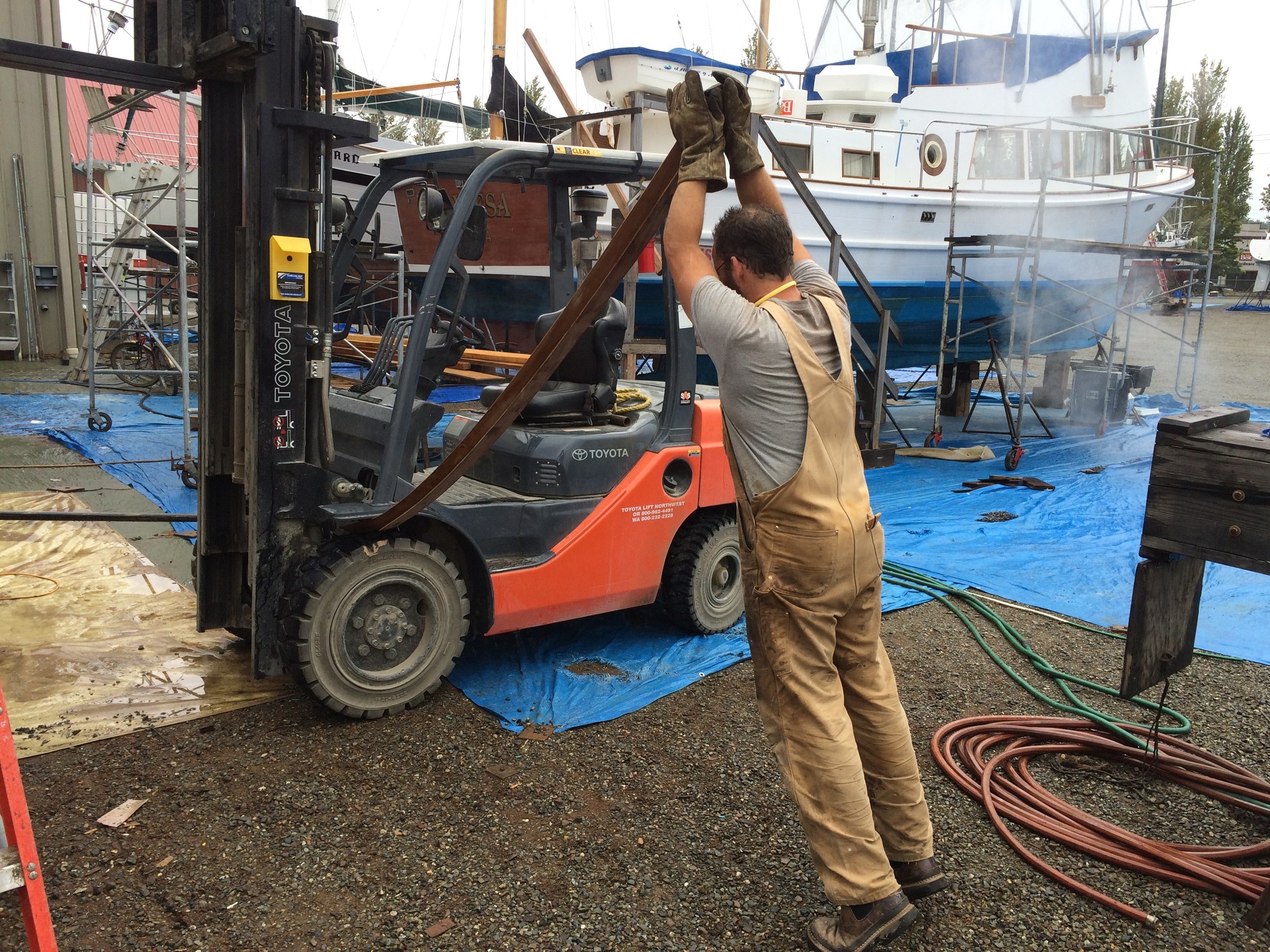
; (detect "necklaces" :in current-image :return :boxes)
[755,281,797,306]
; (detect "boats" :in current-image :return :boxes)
[547,0,1198,377]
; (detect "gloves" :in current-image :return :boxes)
[703,70,768,178]
[666,69,728,193]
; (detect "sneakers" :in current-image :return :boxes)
[890,856,949,901]
[807,888,920,952]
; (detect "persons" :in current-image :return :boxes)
[662,71,946,952]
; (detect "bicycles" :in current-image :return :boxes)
[110,319,178,397]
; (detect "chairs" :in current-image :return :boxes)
[480,295,628,421]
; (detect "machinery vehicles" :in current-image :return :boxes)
[0,1,747,724]
